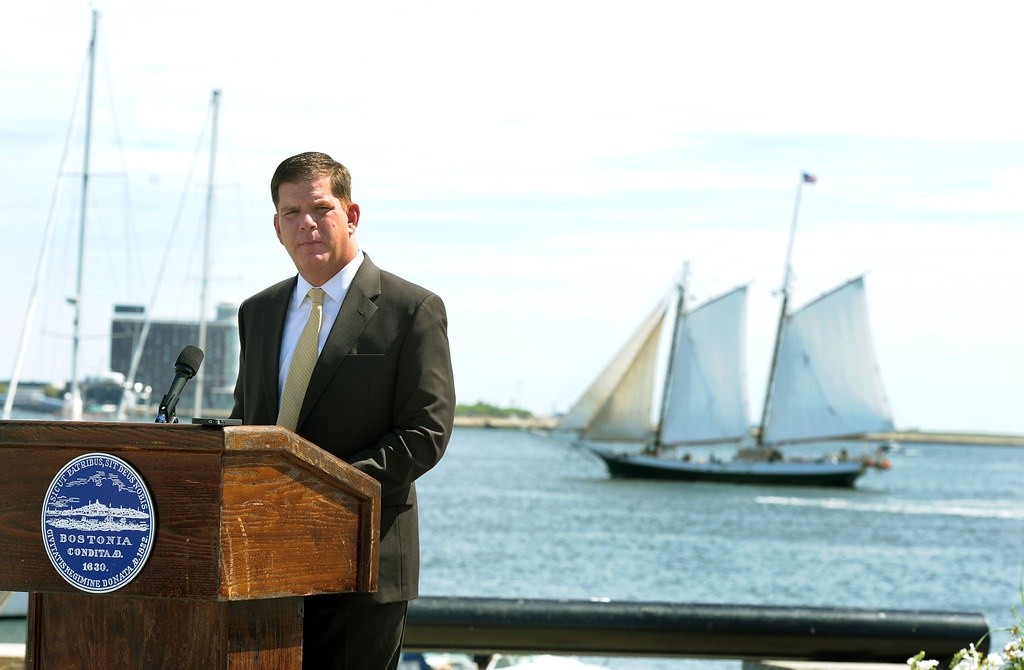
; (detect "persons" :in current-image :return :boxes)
[229,152,456,670]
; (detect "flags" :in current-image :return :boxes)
[802,171,816,183]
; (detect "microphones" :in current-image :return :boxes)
[155,344,205,423]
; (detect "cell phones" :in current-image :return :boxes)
[191,417,242,426]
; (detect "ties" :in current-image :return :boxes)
[276,288,323,432]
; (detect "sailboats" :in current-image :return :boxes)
[582,164,897,489]
[534,289,678,446]
[0,4,222,619]
